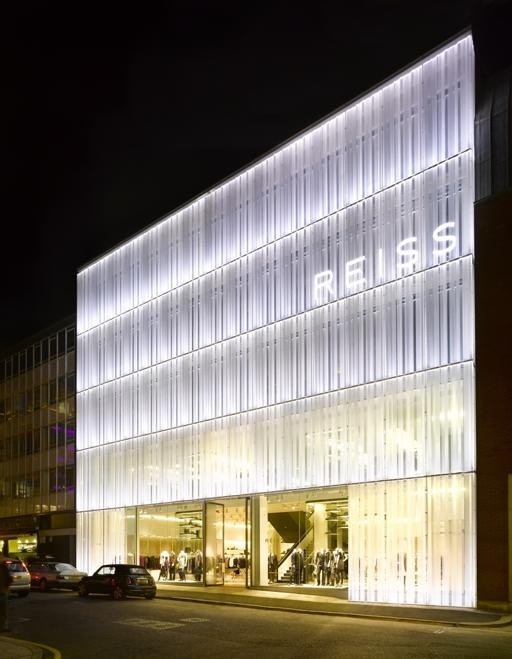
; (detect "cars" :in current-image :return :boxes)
[77,564,157,600]
[27,562,88,591]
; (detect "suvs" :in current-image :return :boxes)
[0,555,31,599]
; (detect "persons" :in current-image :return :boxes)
[295,547,347,588]
[159,548,203,584]
